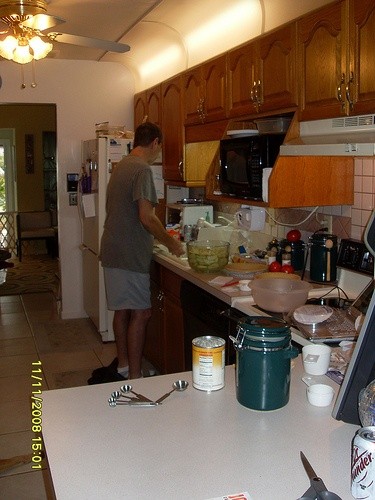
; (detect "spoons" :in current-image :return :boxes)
[107,379,189,407]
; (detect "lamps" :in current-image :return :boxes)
[0,26,54,65]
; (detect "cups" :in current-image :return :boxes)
[278,238,305,271]
[302,345,331,375]
[306,384,333,407]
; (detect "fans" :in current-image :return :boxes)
[0,0,131,54]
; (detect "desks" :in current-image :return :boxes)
[30,343,361,500]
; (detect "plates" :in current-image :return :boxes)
[223,263,269,277]
[226,129,258,138]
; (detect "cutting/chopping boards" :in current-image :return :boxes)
[290,304,364,342]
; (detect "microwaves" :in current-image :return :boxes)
[219,132,286,200]
[167,203,214,238]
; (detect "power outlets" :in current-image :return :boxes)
[322,216,332,235]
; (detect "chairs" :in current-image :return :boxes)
[49,208,58,233]
[16,210,55,262]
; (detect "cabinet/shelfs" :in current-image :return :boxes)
[181,51,258,144]
[141,262,184,375]
[298,0,375,122]
[133,82,161,132]
[161,72,221,188]
[224,16,300,121]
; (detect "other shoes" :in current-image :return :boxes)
[141,367,156,378]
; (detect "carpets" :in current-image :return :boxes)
[0,253,58,295]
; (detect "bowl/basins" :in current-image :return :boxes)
[248,272,313,312]
[186,239,230,273]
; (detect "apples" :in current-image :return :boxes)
[269,261,294,274]
[287,229,301,242]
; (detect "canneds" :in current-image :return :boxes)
[184,224,192,242]
[350,426,375,499]
[191,335,226,392]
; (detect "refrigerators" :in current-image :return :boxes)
[77,138,134,343]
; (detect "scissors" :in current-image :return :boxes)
[296,450,342,500]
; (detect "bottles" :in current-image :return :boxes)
[228,315,300,412]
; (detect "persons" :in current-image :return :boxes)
[100,121,183,380]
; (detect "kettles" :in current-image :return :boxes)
[310,234,337,283]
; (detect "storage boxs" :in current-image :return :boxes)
[95,129,110,138]
[110,125,127,138]
